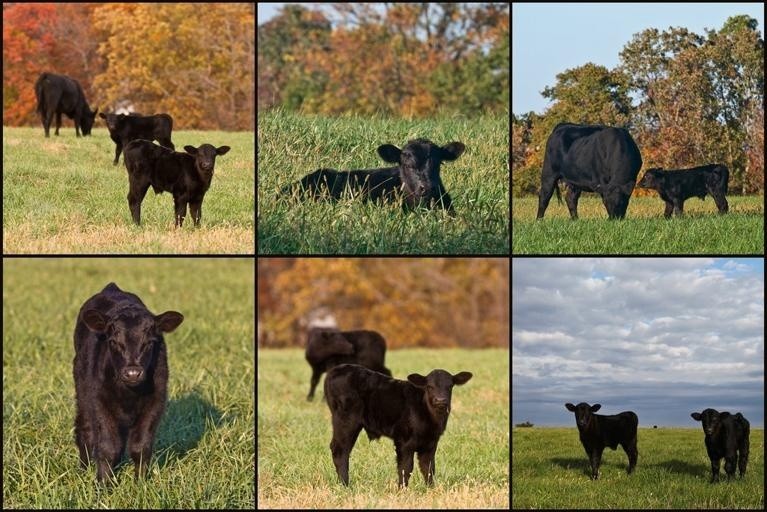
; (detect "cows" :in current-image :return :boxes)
[275,137,466,223]
[564,402,639,481]
[34,72,98,138]
[303,326,393,402]
[690,408,751,485]
[123,138,231,228]
[323,363,473,489]
[98,112,175,166]
[637,163,730,221]
[71,281,185,495]
[535,121,643,221]
